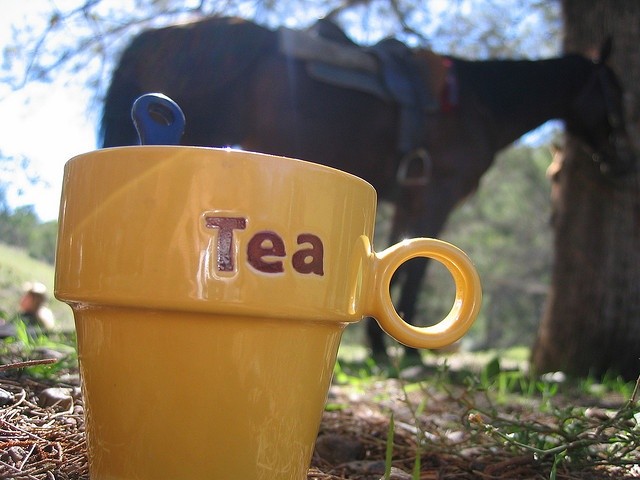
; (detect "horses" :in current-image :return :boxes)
[97,16,633,380]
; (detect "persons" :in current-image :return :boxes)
[0,282,48,340]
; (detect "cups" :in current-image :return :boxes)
[54,145,482,480]
